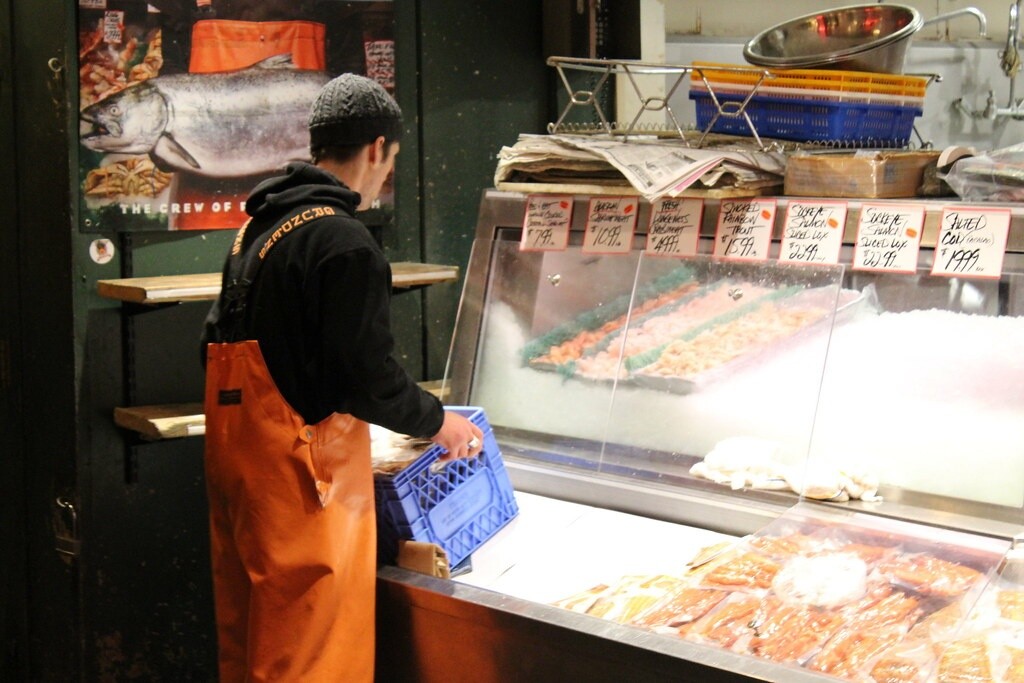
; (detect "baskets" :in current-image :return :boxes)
[689,58,926,148]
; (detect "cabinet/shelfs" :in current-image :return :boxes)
[100,261,459,437]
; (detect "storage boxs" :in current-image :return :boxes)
[689,60,927,143]
[785,151,939,196]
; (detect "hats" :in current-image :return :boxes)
[307,73,405,140]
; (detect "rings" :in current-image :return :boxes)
[468,436,480,447]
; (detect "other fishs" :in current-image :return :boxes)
[78,50,336,177]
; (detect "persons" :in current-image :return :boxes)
[197,73,485,683]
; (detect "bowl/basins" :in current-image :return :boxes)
[742,3,926,79]
